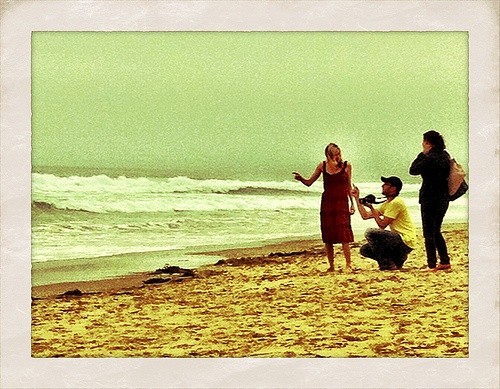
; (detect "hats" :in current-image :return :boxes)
[381,176,402,191]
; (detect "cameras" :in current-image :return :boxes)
[359,194,375,204]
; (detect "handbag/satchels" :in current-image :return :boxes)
[444,150,468,201]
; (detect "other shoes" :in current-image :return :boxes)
[436,263,451,269]
[420,266,437,272]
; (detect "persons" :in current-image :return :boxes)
[352,174,415,272]
[292,142,356,273]
[411,129,453,270]
